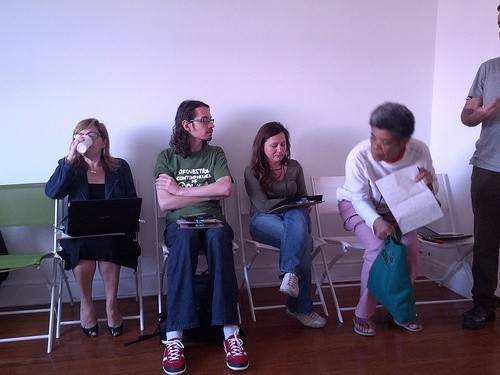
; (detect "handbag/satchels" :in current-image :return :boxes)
[367,235,416,324]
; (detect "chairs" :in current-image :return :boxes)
[412,173,476,305]
[0,182,72,354]
[233,175,329,321]
[152,175,242,326]
[309,175,386,323]
[54,155,145,339]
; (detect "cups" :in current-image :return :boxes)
[76,135,93,153]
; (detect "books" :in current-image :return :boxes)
[266,192,324,213]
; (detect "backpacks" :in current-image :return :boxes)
[158,270,223,340]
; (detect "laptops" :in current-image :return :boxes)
[417,226,474,241]
[64,197,143,237]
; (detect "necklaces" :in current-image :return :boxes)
[88,169,97,174]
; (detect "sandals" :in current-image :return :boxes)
[353,316,376,336]
[388,312,423,332]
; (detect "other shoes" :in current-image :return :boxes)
[81,322,98,336]
[107,321,123,337]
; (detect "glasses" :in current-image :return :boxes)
[74,132,103,140]
[187,118,214,124]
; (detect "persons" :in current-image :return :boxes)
[153,100,255,374]
[45,119,137,338]
[244,122,328,329]
[337,102,438,336]
[461,4,500,332]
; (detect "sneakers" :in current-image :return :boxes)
[286,308,327,328]
[463,306,496,328]
[279,272,299,297]
[223,327,249,370]
[162,338,186,375]
